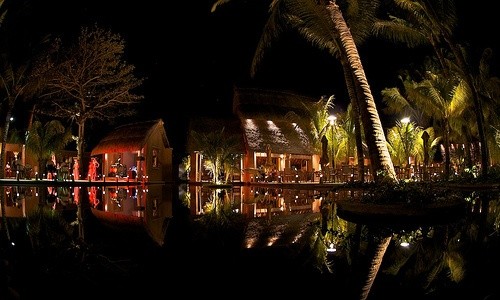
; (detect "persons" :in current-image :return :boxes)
[2,187,139,209]
[5,156,137,180]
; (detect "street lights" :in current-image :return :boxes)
[326,116,338,183]
[400,116,412,181]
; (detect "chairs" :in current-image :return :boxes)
[324,164,441,183]
[284,168,294,182]
[297,169,307,183]
